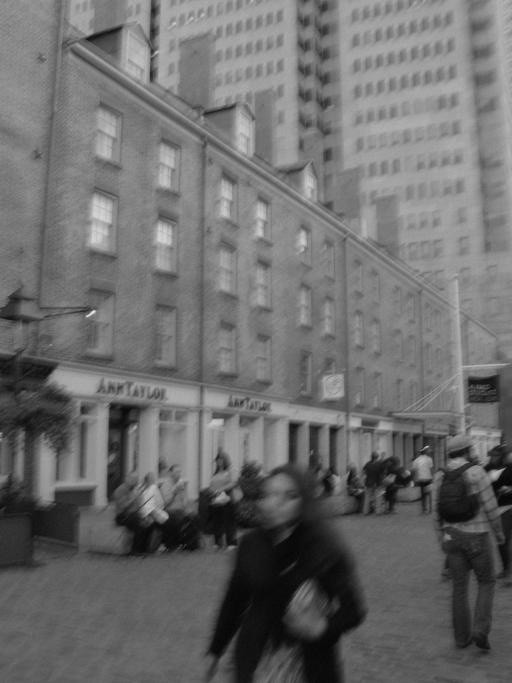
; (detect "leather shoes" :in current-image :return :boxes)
[474,632,490,648]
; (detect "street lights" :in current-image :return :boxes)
[0,284,44,568]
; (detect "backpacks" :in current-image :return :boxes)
[438,462,478,522]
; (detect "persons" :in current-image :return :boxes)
[306,447,435,518]
[483,441,512,583]
[433,435,505,650]
[201,465,367,683]
[115,451,239,553]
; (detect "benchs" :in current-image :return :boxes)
[396,487,422,502]
[313,496,360,517]
[78,505,133,555]
